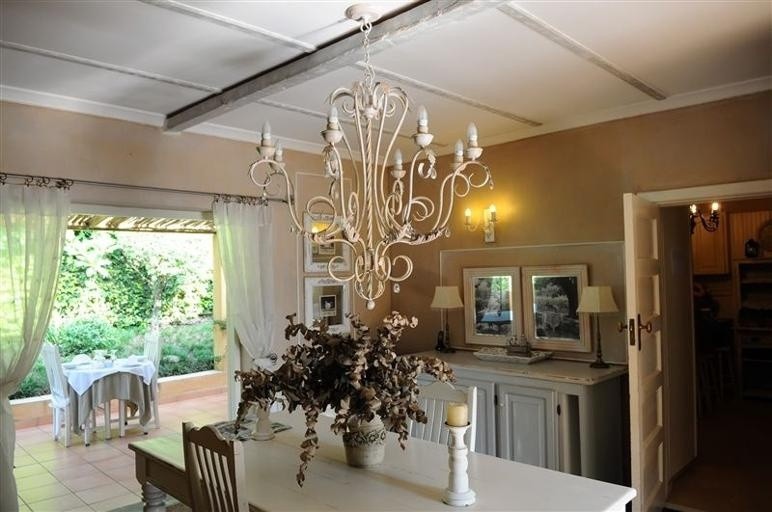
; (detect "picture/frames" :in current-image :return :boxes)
[461,267,524,347]
[304,276,352,332]
[521,263,592,353]
[303,212,351,274]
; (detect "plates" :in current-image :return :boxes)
[122,363,140,367]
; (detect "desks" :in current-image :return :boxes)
[128,405,637,512]
[62,359,156,440]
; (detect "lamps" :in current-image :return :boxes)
[245,4,493,313]
[464,205,496,245]
[690,201,721,233]
[430,285,464,353]
[575,284,624,368]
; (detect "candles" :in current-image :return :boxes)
[446,401,470,426]
[261,118,273,146]
[417,105,429,133]
[467,121,479,147]
[454,139,463,155]
[394,147,403,169]
[465,207,472,224]
[272,137,283,161]
[327,104,339,130]
[489,204,497,221]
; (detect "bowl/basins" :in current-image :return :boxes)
[62,363,77,369]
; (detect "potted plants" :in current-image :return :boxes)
[234,307,457,489]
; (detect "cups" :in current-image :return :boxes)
[128,355,137,363]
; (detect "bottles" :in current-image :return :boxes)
[104,355,113,368]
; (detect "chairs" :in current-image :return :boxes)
[182,421,250,511]
[113,331,163,438]
[408,381,477,450]
[39,342,112,447]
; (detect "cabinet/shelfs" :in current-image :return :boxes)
[403,365,560,478]
[735,261,771,428]
